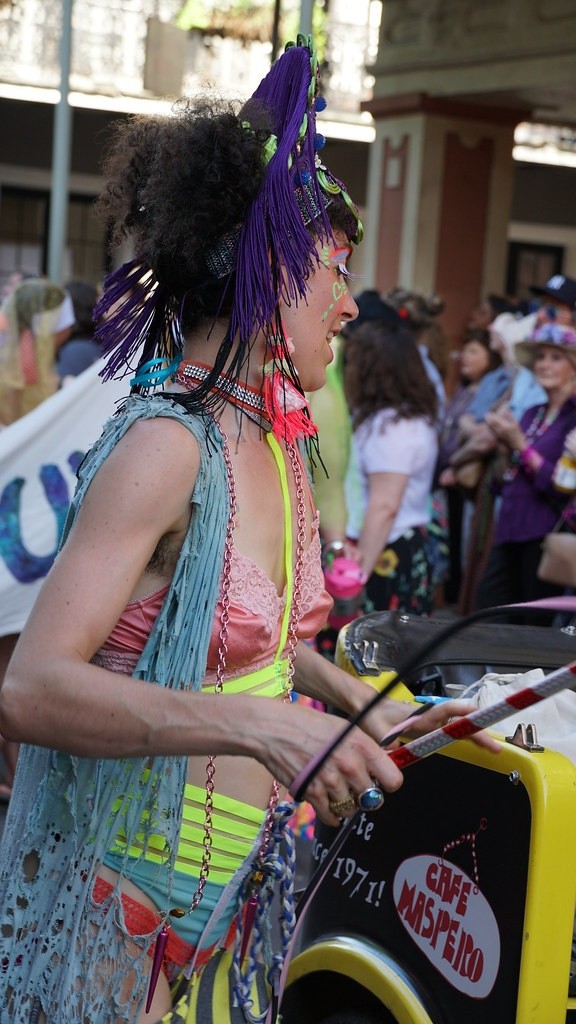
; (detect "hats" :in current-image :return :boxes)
[512,322,576,371]
[528,274,576,310]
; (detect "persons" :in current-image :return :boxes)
[0,95,501,1024]
[306,271,575,616]
[0,266,148,429]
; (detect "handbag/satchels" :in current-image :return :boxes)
[460,668,576,767]
[537,532,576,587]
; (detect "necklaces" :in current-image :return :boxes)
[177,357,276,435]
[503,399,561,480]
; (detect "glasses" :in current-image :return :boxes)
[530,299,557,320]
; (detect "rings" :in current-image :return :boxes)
[357,780,384,811]
[330,794,356,813]
